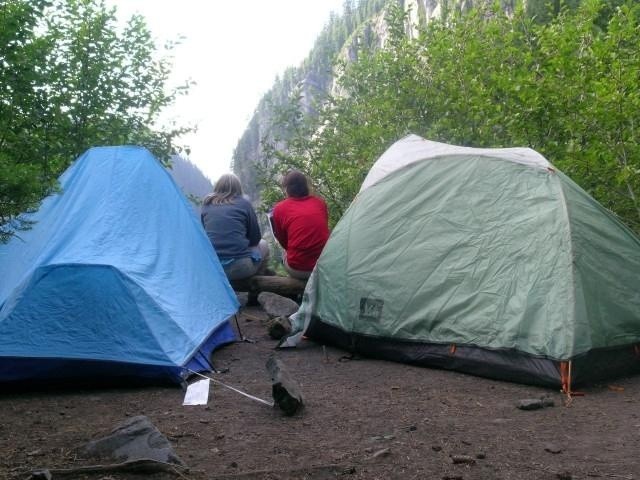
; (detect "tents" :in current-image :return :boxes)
[1,142,243,387]
[279,132,639,395]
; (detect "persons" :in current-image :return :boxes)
[199,173,270,306]
[269,171,332,279]
